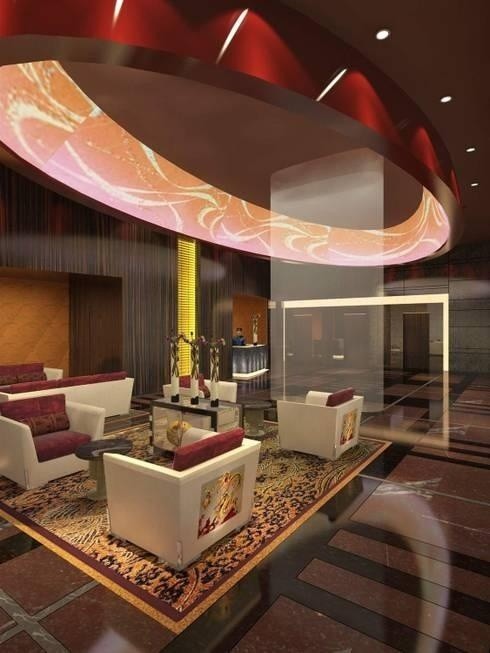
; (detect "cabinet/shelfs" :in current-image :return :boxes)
[149,395,244,459]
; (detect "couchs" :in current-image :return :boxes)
[0,363,137,418]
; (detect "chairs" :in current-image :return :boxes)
[275,389,366,463]
[100,426,263,572]
[0,394,108,491]
[160,376,237,402]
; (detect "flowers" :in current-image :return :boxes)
[160,328,228,381]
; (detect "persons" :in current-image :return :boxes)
[231,328,245,355]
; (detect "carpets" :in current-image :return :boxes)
[0,415,392,633]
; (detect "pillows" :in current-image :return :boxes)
[0,392,70,438]
[326,386,355,405]
[179,373,211,398]
[172,426,246,471]
